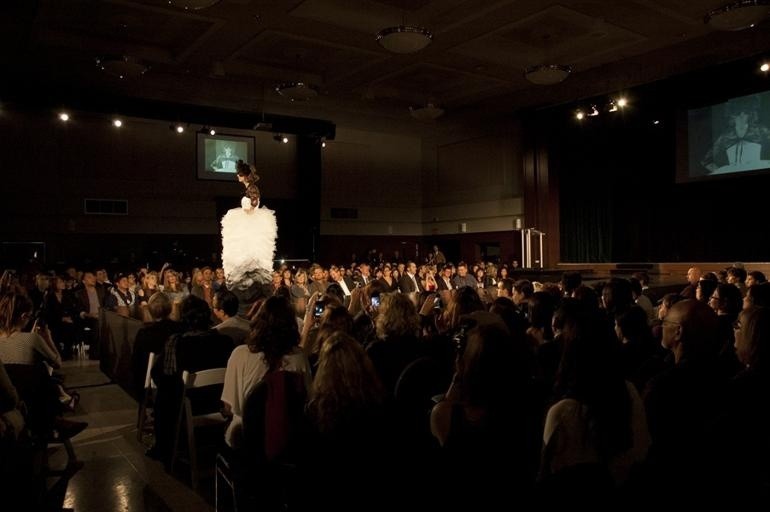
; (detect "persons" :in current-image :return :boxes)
[0,244,770,511]
[219,160,280,287]
[210,145,243,172]
[700,94,769,174]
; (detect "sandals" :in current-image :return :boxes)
[67,391,79,411]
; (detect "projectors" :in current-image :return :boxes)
[253,122,272,131]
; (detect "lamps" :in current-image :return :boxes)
[98,57,146,85]
[703,0,770,33]
[376,25,433,54]
[274,80,321,99]
[524,65,569,85]
[409,104,445,121]
[57,105,336,150]
